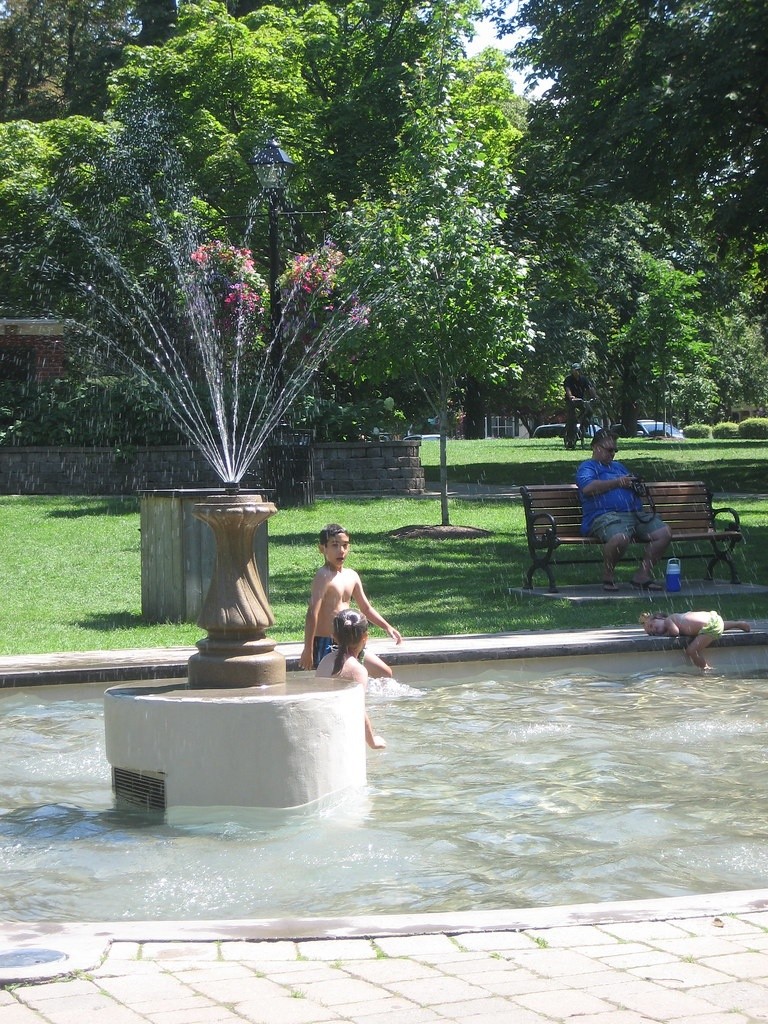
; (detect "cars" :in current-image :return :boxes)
[402,433,450,441]
[637,420,685,440]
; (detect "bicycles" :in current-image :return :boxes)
[563,398,599,449]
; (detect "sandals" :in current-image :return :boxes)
[601,580,618,592]
[629,579,662,591]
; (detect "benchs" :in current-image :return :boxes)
[517,481,744,594]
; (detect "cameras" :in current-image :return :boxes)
[630,473,645,496]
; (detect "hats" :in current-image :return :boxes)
[572,363,581,369]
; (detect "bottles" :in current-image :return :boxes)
[666,558,681,591]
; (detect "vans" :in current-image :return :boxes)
[533,421,601,438]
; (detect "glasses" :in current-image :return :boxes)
[598,444,618,453]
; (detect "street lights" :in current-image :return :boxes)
[248,135,318,508]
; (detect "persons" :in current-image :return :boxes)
[297,525,402,677]
[563,362,601,447]
[639,610,751,669]
[576,429,672,591]
[316,609,386,749]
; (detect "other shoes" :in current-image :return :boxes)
[568,442,573,450]
